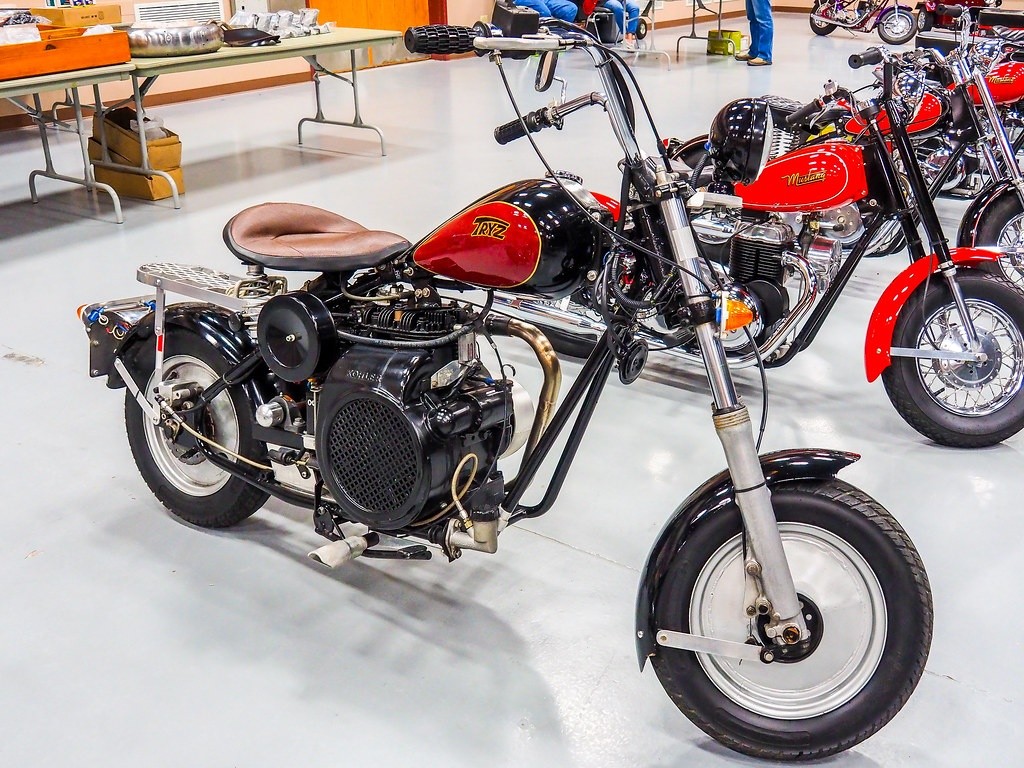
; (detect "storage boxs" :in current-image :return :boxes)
[93,165,186,201]
[93,106,182,169]
[87,136,180,173]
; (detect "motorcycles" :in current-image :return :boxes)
[77,0,1024,765]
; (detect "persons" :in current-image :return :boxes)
[735,0,774,66]
[513,0,578,31]
[582,0,639,51]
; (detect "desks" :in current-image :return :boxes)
[94,26,404,210]
[0,63,135,224]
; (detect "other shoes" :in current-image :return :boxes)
[620,39,640,52]
[747,56,772,65]
[735,54,755,61]
